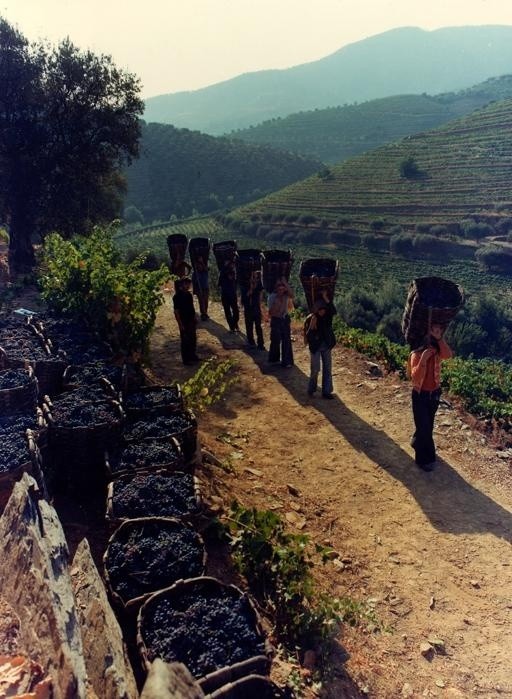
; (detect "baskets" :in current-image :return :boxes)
[203,674,282,697]
[135,575,274,685]
[212,240,237,270]
[189,238,210,271]
[298,259,337,310]
[233,248,262,283]
[260,251,292,290]
[1,315,207,607]
[401,276,465,346]
[167,234,188,261]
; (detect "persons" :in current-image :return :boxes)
[239,266,268,350]
[264,276,297,369]
[190,255,211,322]
[167,254,194,279]
[407,323,454,474]
[302,288,340,399]
[217,259,241,334]
[172,275,201,368]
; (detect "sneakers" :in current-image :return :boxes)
[200,312,335,402]
[417,461,434,472]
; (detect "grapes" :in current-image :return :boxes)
[0,310,265,681]
